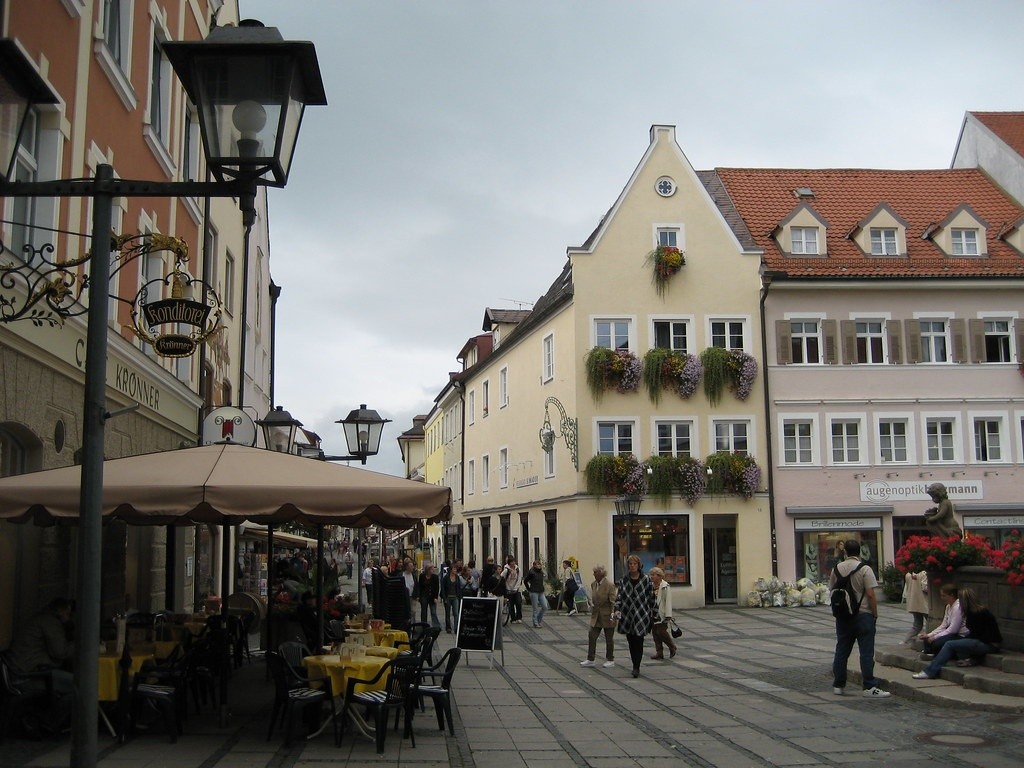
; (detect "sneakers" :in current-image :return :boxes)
[580,660,595,667]
[833,686,843,695]
[602,660,614,668]
[863,687,891,698]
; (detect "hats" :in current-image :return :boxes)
[301,591,318,601]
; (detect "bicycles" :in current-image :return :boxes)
[502,588,520,627]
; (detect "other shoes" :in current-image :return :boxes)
[447,629,451,633]
[538,623,544,627]
[632,669,640,677]
[532,624,541,628]
[920,654,936,661]
[670,645,677,658]
[912,671,930,679]
[516,620,522,624]
[650,654,663,659]
[569,609,576,615]
[959,659,978,667]
[511,621,516,624]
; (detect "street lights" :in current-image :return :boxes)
[254,404,392,629]
[612,490,643,576]
[0,20,327,768]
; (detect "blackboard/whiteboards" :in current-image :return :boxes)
[456,596,503,653]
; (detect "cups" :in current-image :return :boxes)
[341,642,367,658]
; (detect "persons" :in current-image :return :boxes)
[324,536,358,584]
[284,552,317,583]
[8,597,75,739]
[985,537,994,550]
[524,560,548,628]
[926,482,962,539]
[614,554,658,677]
[363,554,522,634]
[562,560,577,615]
[579,564,617,668]
[648,567,677,659]
[825,540,846,572]
[919,583,970,661]
[912,587,1003,679]
[899,569,928,645]
[829,539,891,698]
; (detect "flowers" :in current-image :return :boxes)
[586,450,763,509]
[643,345,702,412]
[895,529,1024,591]
[272,584,352,616]
[642,233,688,301]
[582,346,644,410]
[698,347,758,409]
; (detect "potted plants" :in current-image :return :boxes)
[879,561,903,602]
[539,544,565,610]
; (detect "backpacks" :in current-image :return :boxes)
[831,563,870,619]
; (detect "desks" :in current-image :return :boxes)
[60,652,155,738]
[322,645,399,661]
[304,655,391,742]
[192,612,219,622]
[345,628,410,651]
[160,622,204,640]
[129,641,185,660]
[343,620,391,629]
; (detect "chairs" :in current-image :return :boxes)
[0,608,462,754]
[370,563,413,626]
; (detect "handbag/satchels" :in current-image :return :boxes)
[566,568,579,593]
[671,628,682,638]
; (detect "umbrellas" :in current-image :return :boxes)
[0,437,450,728]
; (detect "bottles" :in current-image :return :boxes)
[367,624,372,632]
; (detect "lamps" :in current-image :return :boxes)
[539,397,579,472]
[854,473,865,479]
[886,472,899,478]
[705,466,713,474]
[952,471,965,477]
[647,465,652,474]
[919,472,932,478]
[984,471,998,476]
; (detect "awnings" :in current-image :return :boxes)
[243,528,328,549]
